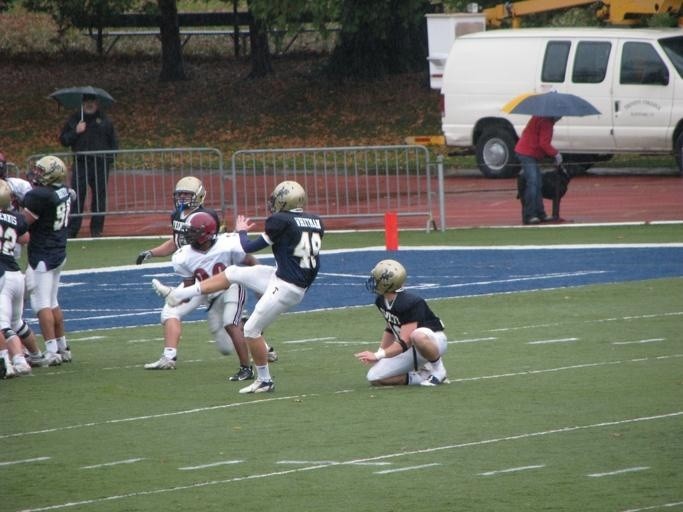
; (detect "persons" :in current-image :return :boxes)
[0,151,77,381]
[170,210,277,381]
[57,92,120,238]
[134,176,233,370]
[352,259,452,387]
[512,115,563,225]
[150,179,323,394]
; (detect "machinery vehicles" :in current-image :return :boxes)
[404,0,682,156]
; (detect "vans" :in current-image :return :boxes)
[439,26,682,178]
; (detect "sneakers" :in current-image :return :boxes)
[238,378,275,394]
[144,354,177,371]
[228,365,255,381]
[423,363,449,385]
[268,347,278,361]
[420,368,446,387]
[4,345,71,379]
[151,278,182,307]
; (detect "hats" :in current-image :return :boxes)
[83,94,95,101]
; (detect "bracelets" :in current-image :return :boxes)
[373,349,386,361]
[376,347,384,353]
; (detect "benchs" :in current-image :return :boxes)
[84,12,342,55]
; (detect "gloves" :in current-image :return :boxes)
[135,252,152,265]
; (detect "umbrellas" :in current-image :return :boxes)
[47,85,115,120]
[509,90,602,120]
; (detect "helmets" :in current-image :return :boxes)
[170,175,208,212]
[32,154,67,187]
[0,153,9,181]
[179,211,217,250]
[265,180,305,215]
[365,259,405,294]
[0,179,13,213]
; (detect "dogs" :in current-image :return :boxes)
[516,161,587,222]
